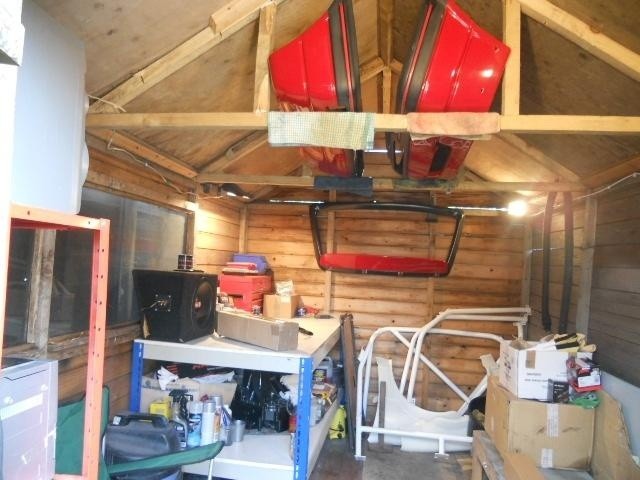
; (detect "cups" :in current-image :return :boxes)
[289,415,296,434]
[178,254,193,270]
[222,428,232,446]
[230,420,246,442]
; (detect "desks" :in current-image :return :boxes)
[470,430,595,479]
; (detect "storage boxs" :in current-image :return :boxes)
[217,311,300,350]
[262,294,300,319]
[500,339,592,400]
[484,376,595,471]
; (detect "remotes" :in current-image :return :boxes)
[298,327,313,337]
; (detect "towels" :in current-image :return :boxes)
[406,112,500,141]
[268,111,374,150]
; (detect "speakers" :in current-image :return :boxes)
[132,269,218,342]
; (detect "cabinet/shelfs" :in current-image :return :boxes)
[129,316,343,480]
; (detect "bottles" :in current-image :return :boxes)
[168,392,223,451]
